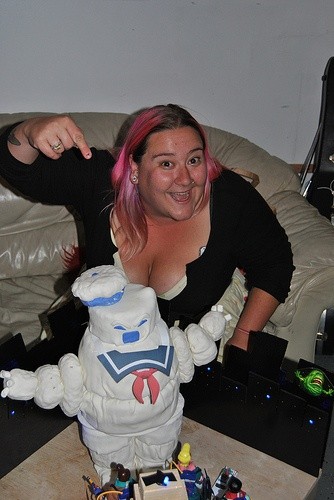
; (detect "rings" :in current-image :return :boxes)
[50,142,62,150]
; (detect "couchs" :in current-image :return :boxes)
[1,112,334,364]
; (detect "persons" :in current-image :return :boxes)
[0,104,296,351]
